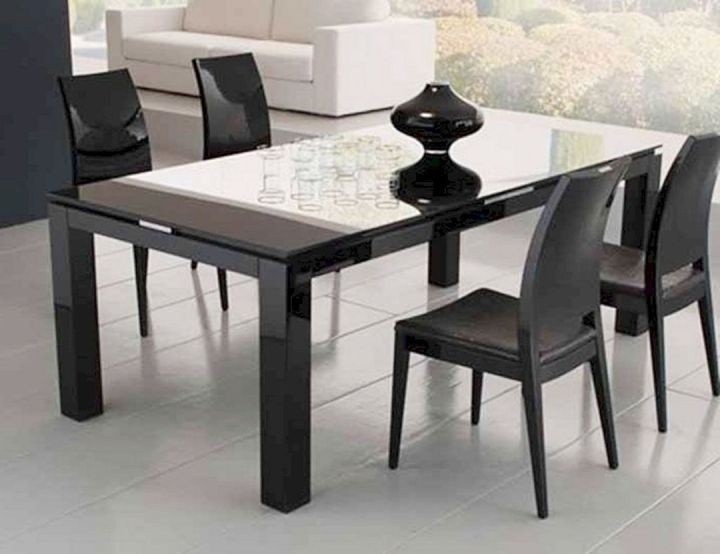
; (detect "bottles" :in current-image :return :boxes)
[389,150,481,215]
[390,83,483,150]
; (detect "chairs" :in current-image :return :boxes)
[58,68,229,336]
[389,156,631,520]
[191,51,343,274]
[587,129,720,432]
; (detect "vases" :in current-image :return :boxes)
[389,84,484,138]
[388,152,482,213]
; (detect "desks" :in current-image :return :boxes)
[45,108,688,513]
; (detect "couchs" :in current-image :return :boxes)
[106,0,435,115]
